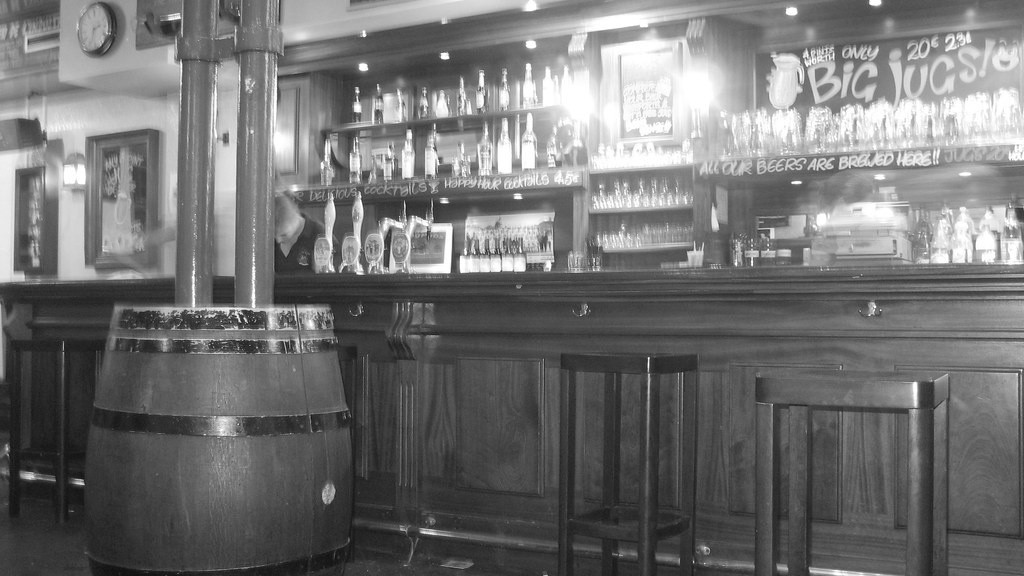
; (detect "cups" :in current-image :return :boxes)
[730,231,791,266]
[715,88,1021,157]
[567,140,703,270]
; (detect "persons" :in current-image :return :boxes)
[274,197,339,272]
[466,228,552,254]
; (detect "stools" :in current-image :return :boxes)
[556,351,699,576]
[754,369,949,576]
[9,338,104,525]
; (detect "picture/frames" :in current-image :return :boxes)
[13,166,59,272]
[84,128,159,268]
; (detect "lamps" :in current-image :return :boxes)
[62,152,88,194]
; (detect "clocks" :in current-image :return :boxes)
[76,2,118,59]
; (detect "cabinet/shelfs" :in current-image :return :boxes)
[272,70,1024,270]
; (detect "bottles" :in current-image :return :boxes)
[912,204,1023,264]
[320,63,573,183]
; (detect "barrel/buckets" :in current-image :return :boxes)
[86,303,354,576]
